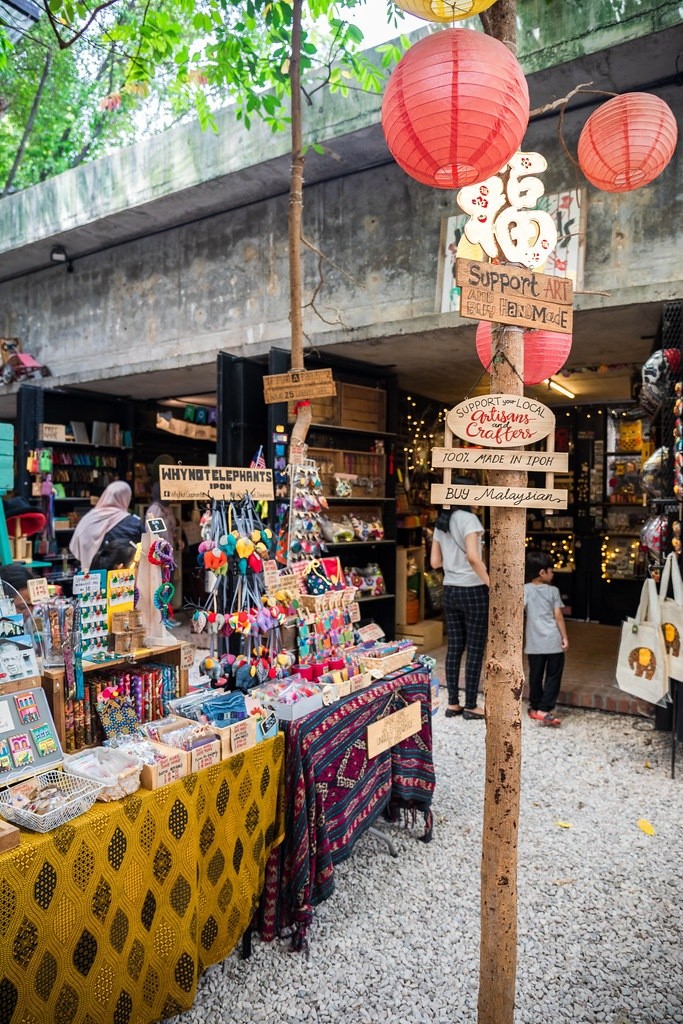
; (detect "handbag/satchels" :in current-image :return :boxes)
[615,577,669,708]
[646,551,682,682]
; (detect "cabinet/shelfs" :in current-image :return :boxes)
[523,403,654,628]
[13,385,138,570]
[292,420,400,644]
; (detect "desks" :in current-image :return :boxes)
[1,643,286,1024]
[192,653,436,955]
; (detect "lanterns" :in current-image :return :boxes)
[388,0,502,26]
[475,320,573,387]
[380,26,530,193]
[578,89,679,191]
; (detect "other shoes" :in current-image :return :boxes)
[462,706,485,721]
[445,707,464,718]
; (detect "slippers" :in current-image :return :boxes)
[529,709,537,719]
[536,710,562,724]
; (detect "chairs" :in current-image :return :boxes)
[0,337,23,382]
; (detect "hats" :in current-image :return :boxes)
[3,495,47,538]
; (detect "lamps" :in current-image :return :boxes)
[49,244,66,263]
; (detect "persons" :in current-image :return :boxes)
[521,553,571,726]
[146,484,188,629]
[0,563,38,633]
[94,540,138,576]
[431,477,491,721]
[68,480,146,574]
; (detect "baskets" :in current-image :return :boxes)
[0,770,107,833]
[353,645,417,676]
[299,585,358,614]
